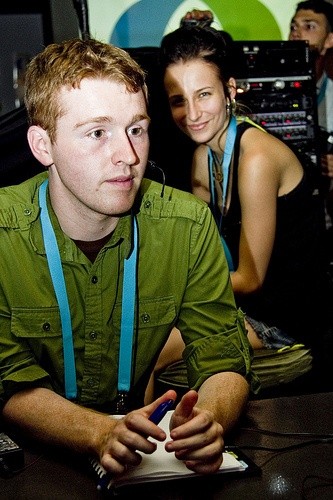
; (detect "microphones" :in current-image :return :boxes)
[148,160,165,197]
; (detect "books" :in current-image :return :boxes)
[89,409,246,492]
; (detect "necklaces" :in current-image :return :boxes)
[210,150,224,195]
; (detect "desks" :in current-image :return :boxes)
[0,393,333,500]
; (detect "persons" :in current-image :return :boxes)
[141,10,333,407]
[185,0,333,144]
[0,36,264,476]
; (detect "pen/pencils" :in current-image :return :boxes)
[95,399,175,490]
